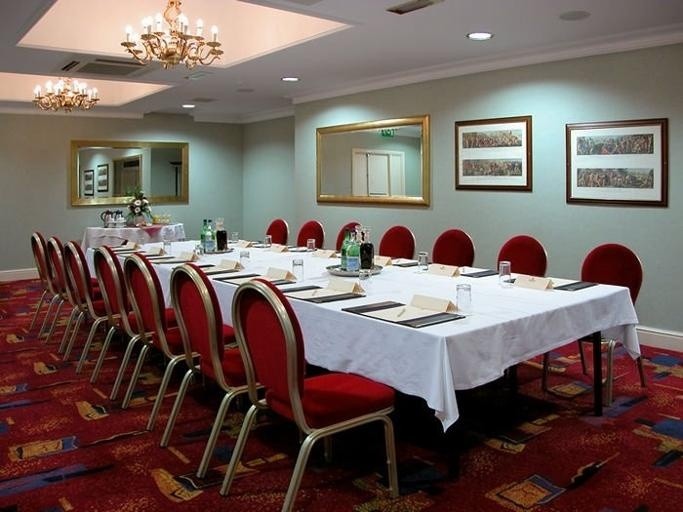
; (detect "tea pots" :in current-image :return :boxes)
[100,210,113,228]
[113,210,123,225]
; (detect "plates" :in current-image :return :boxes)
[326,264,383,277]
[203,248,234,255]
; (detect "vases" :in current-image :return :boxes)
[130,214,147,226]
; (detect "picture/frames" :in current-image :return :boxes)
[95,163,109,193]
[565,117,670,209]
[82,169,95,197]
[453,113,534,194]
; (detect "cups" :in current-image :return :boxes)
[358,269,371,291]
[456,284,473,314]
[418,252,428,273]
[232,232,239,242]
[292,259,304,281]
[264,235,272,247]
[499,260,511,286]
[137,239,144,248]
[195,243,204,259]
[240,251,249,268]
[307,239,315,253]
[164,240,172,255]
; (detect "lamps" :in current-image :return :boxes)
[466,30,495,42]
[31,77,102,115]
[120,0,224,72]
[279,76,301,82]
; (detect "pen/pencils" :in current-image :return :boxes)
[311,289,318,295]
[397,307,405,317]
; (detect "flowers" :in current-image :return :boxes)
[124,181,155,224]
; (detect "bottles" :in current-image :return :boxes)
[205,219,215,253]
[341,228,350,271]
[201,219,208,254]
[355,226,363,244]
[346,230,361,273]
[360,226,374,269]
[215,217,227,251]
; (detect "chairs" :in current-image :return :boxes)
[160,261,310,481]
[265,216,290,246]
[295,218,326,248]
[335,221,362,254]
[29,229,100,340]
[121,250,239,433]
[377,223,418,263]
[36,234,103,356]
[89,244,180,402]
[430,227,476,268]
[57,240,109,376]
[541,241,647,408]
[495,233,549,278]
[218,278,400,512]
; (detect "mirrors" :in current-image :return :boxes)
[69,137,192,208]
[315,113,432,208]
